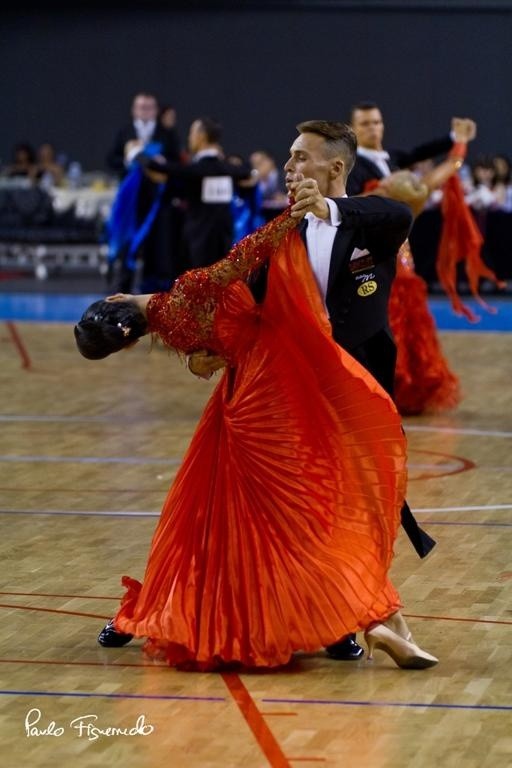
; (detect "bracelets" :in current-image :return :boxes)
[449,143,466,158]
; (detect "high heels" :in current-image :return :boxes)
[365,621,440,670]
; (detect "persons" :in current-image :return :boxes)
[120,115,236,271]
[101,141,267,271]
[106,91,181,296]
[343,100,479,196]
[223,154,260,202]
[95,116,441,663]
[486,151,512,212]
[249,148,286,201]
[68,170,443,679]
[30,140,68,188]
[158,101,189,165]
[5,141,38,179]
[352,116,510,418]
[470,158,506,210]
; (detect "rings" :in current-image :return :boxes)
[306,197,311,204]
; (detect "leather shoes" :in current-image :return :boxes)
[97,617,134,647]
[325,633,364,661]
